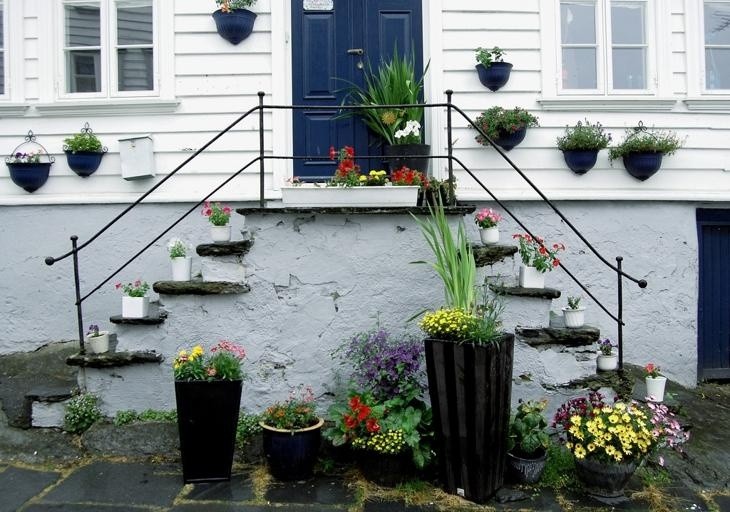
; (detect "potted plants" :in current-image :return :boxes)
[62,121,109,178]
[608,120,679,182]
[168,240,193,282]
[557,118,614,175]
[474,46,513,92]
[425,174,458,208]
[562,296,586,329]
[328,36,432,175]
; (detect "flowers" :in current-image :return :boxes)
[352,428,412,455]
[284,144,431,188]
[6,150,41,164]
[473,208,505,229]
[172,340,245,383]
[642,362,661,381]
[85,324,104,337]
[115,280,150,297]
[550,392,692,470]
[263,385,319,432]
[201,201,232,227]
[511,397,552,457]
[382,111,422,145]
[418,273,508,347]
[512,233,565,273]
[215,0,257,15]
[468,105,540,146]
[597,337,619,356]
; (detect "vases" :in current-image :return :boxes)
[212,8,257,45]
[5,129,56,192]
[519,265,546,289]
[258,416,324,483]
[597,350,619,372]
[506,446,549,486]
[174,379,244,484]
[212,224,231,242]
[87,330,110,354]
[645,375,667,403]
[479,227,501,245]
[491,126,526,152]
[122,295,150,319]
[571,448,646,498]
[422,332,517,504]
[367,452,408,489]
